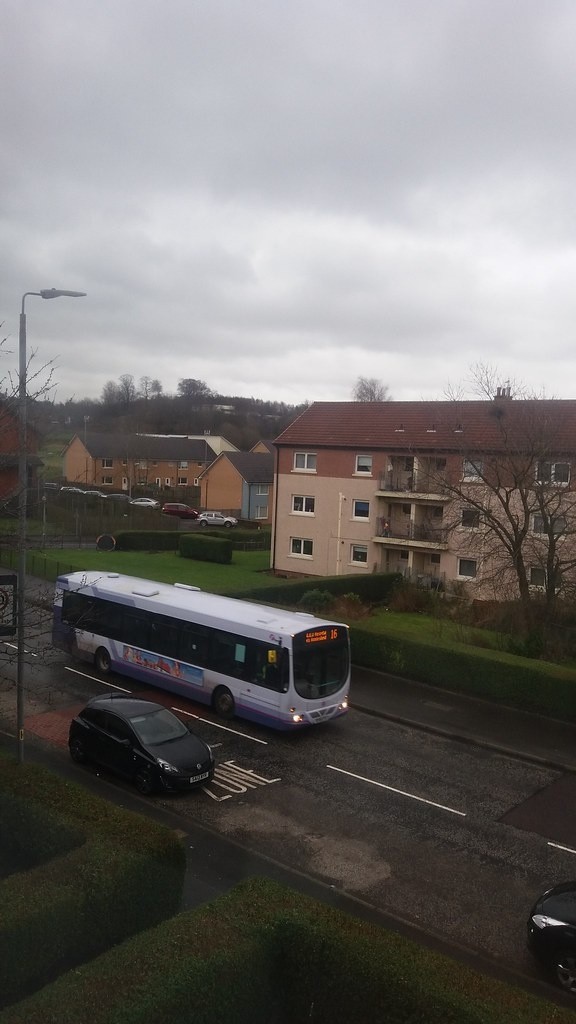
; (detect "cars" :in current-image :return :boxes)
[130,498,161,510]
[197,511,238,527]
[45,482,133,501]
[161,504,200,519]
[528,881,576,994]
[69,691,215,795]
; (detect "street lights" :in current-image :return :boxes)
[126,432,138,489]
[336,494,347,576]
[38,452,52,501]
[130,463,139,500]
[15,289,86,764]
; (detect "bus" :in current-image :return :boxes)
[51,567,352,733]
